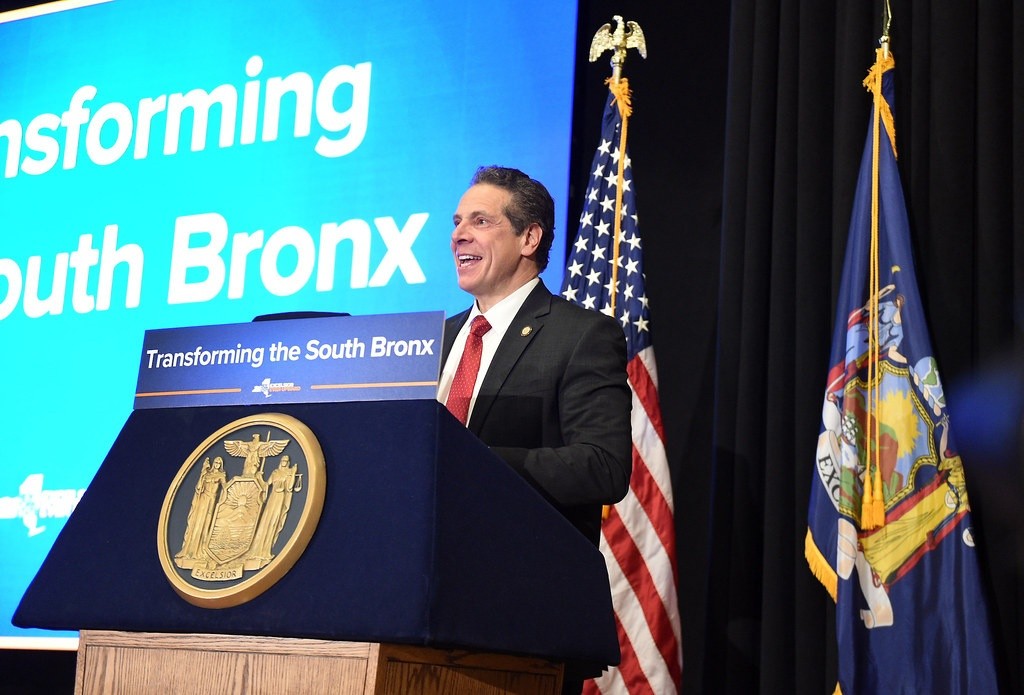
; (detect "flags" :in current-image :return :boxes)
[806,47,999,694]
[559,73,685,695]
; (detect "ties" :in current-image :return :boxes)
[444,313,491,426]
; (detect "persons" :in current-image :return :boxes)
[436,164,633,694]
